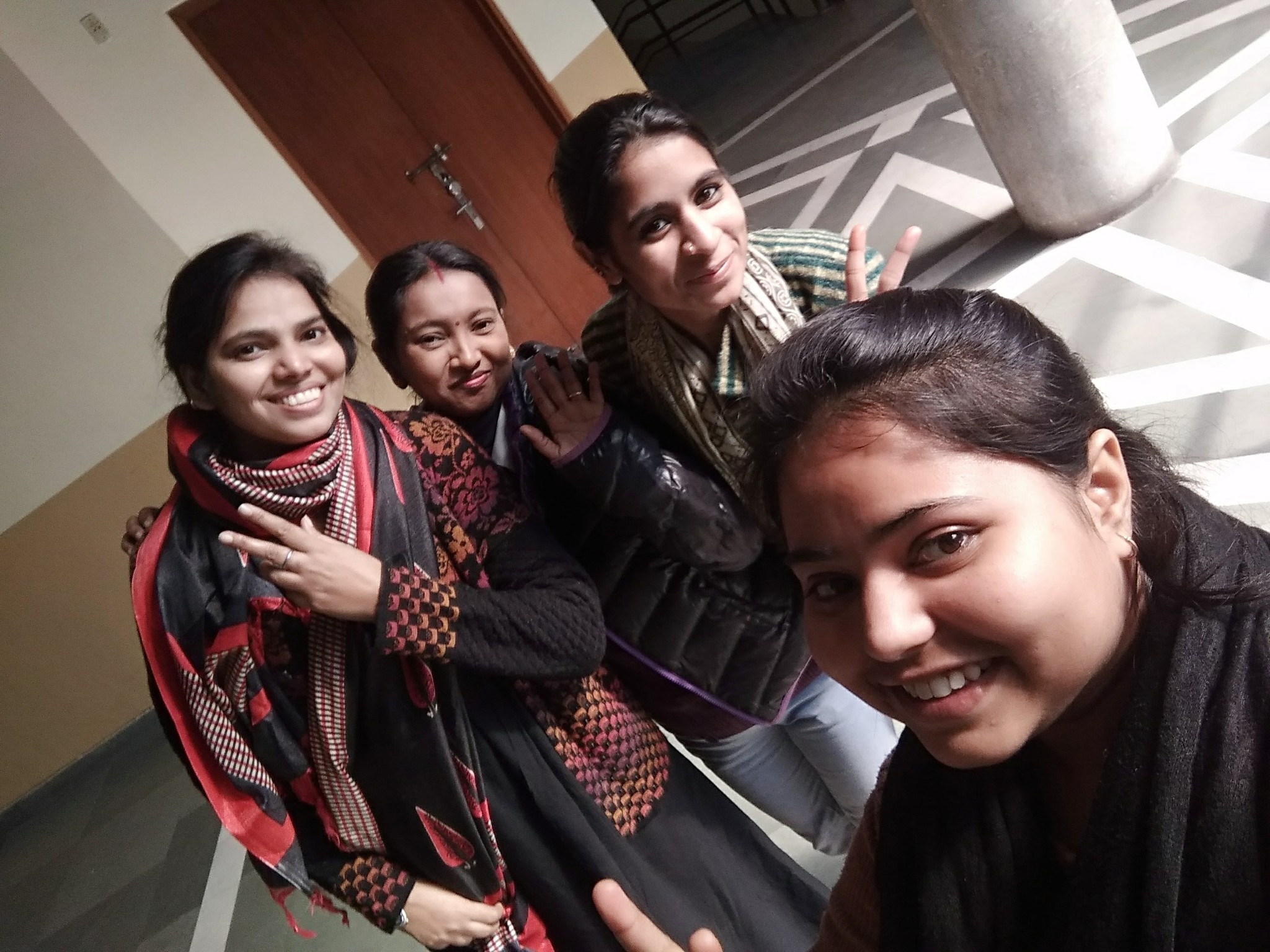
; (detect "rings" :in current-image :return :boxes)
[568,390,585,399]
[281,548,294,569]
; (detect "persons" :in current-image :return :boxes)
[131,230,833,952]
[547,93,923,558]
[119,239,899,857]
[590,284,1269,952]
